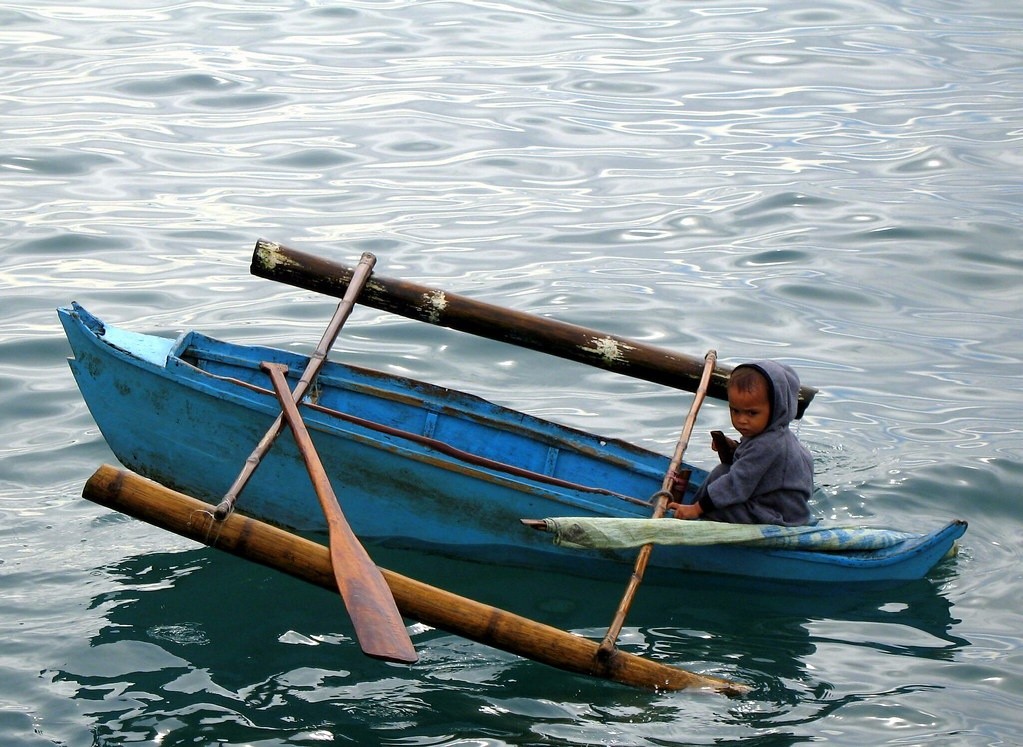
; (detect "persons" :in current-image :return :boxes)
[667,360,814,527]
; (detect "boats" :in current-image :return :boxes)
[54,233,970,700]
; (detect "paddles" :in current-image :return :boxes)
[259,360,420,665]
